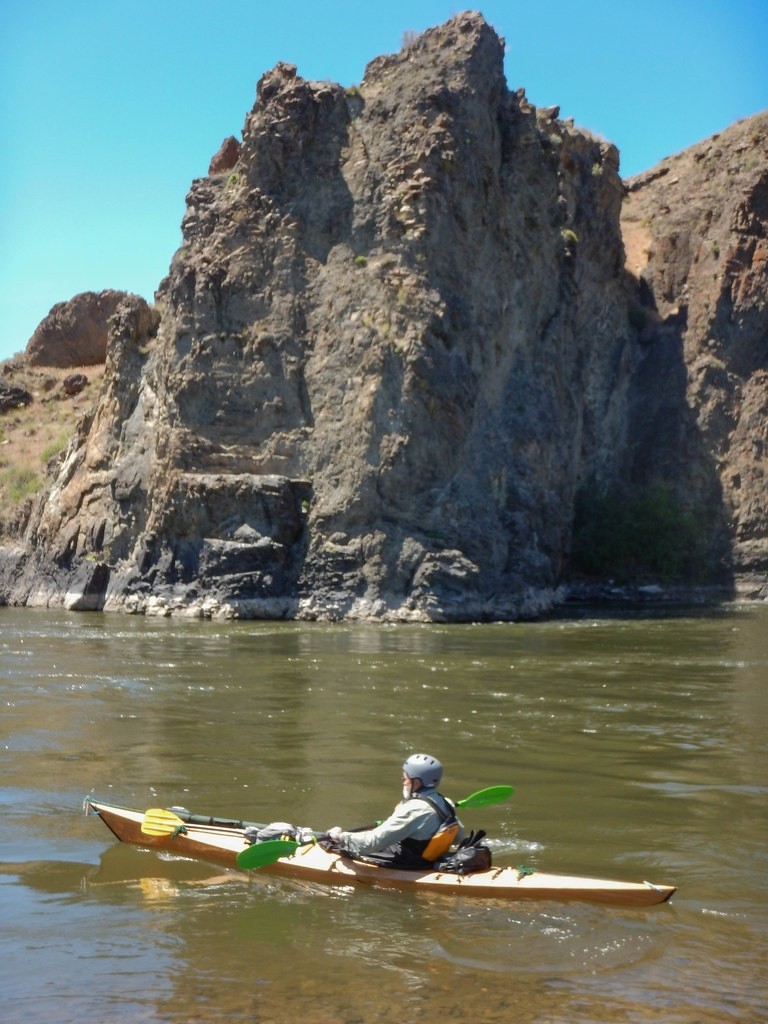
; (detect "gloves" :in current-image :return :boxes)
[327,827,343,841]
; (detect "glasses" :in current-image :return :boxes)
[400,777,410,783]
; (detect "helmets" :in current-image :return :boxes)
[402,753,442,788]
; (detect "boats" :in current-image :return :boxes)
[84,796,678,906]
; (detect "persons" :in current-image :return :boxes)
[326,753,463,873]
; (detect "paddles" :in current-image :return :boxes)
[235,784,515,870]
[140,808,243,837]
